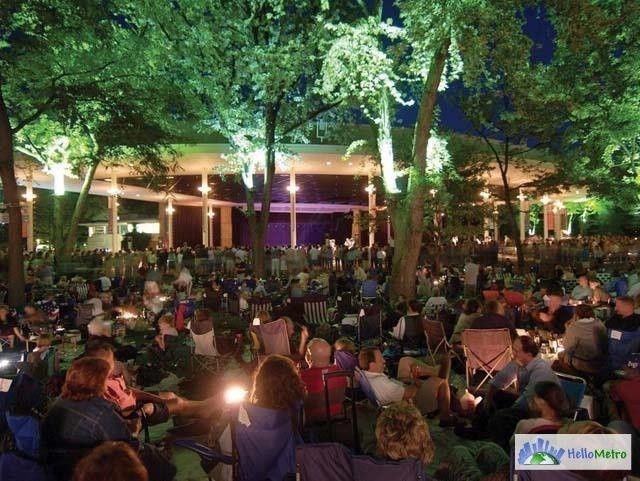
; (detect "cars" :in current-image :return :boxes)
[570,301,582,306]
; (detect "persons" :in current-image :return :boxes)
[0,235,640,480]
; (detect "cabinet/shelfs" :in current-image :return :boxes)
[142,308,147,319]
[461,389,475,419]
[533,327,563,354]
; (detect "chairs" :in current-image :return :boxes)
[0,232,639,480]
